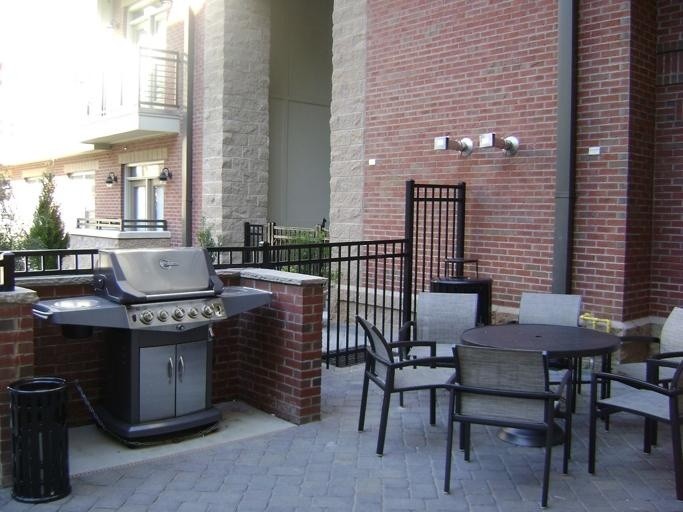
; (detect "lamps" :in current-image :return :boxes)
[105,171,118,188]
[432,132,520,157]
[158,167,172,185]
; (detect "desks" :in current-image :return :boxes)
[460,323,621,477]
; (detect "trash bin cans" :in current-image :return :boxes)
[5,375,71,505]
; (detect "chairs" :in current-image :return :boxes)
[605,308,683,444]
[408,292,479,426]
[444,343,576,507]
[520,291,582,329]
[588,351,682,501]
[356,315,456,456]
